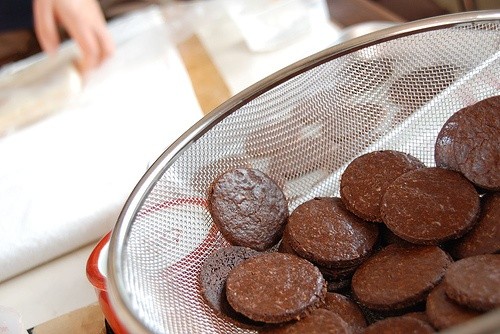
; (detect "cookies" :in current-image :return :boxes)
[198,97,500,334]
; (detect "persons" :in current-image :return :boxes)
[0,0,115,75]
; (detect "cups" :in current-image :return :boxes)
[86,198,231,334]
